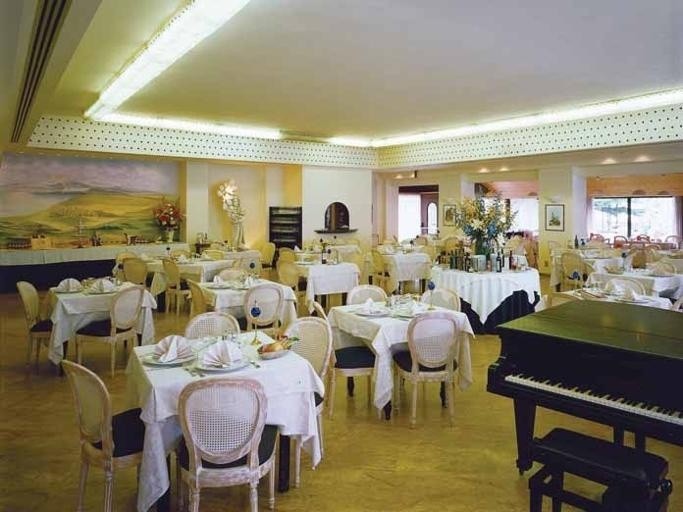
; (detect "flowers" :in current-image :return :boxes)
[153,197,186,231]
[447,191,519,241]
[217,178,248,224]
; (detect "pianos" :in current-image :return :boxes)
[486,300,682,477]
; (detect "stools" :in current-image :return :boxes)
[529,426,674,511]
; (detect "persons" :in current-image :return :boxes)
[226,191,248,252]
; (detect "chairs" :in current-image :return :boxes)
[548,232,681,310]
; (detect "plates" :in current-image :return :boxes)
[142,352,247,375]
[204,282,247,291]
[55,287,115,296]
[615,295,649,305]
[355,307,418,319]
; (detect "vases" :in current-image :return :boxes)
[231,222,246,249]
[164,229,174,243]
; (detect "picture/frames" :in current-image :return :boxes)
[545,203,565,232]
[442,206,456,227]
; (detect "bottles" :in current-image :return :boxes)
[621,253,628,269]
[444,247,514,273]
[320,246,327,264]
[574,235,578,248]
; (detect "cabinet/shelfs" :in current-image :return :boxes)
[268,205,304,250]
